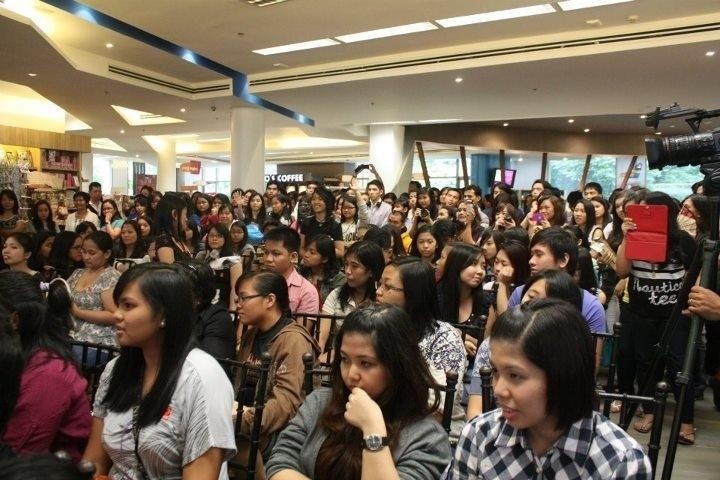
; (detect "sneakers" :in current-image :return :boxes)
[610,399,622,413]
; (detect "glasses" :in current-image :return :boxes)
[342,206,356,210]
[458,199,473,205]
[206,234,224,239]
[374,280,404,292]
[234,292,263,304]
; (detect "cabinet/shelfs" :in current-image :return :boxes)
[7,144,83,225]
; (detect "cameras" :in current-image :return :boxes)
[355,164,369,173]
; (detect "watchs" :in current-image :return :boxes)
[358,433,392,451]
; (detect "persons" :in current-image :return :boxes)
[28,200,60,234]
[690,179,718,199]
[61,230,123,371]
[182,218,198,248]
[682,281,720,324]
[75,221,98,240]
[374,255,469,424]
[99,199,123,240]
[65,191,102,232]
[439,245,484,366]
[1,189,27,234]
[240,228,316,357]
[111,219,143,257]
[192,180,362,229]
[388,211,503,261]
[452,299,656,479]
[80,262,238,479]
[465,267,604,430]
[364,228,396,266]
[391,180,624,226]
[264,303,454,479]
[44,232,83,268]
[675,192,714,238]
[357,179,391,227]
[482,239,531,297]
[86,181,106,230]
[193,221,232,261]
[170,259,234,382]
[123,185,162,218]
[228,221,256,255]
[1,236,41,281]
[134,216,153,243]
[613,191,645,419]
[154,195,194,268]
[613,191,700,446]
[33,233,56,267]
[229,271,322,443]
[301,227,346,298]
[505,226,603,388]
[319,239,386,363]
[0,269,95,479]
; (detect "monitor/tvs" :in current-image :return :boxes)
[491,168,516,188]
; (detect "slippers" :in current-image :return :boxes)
[677,426,696,445]
[636,418,653,432]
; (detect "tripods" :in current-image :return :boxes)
[605,233,720,480]
